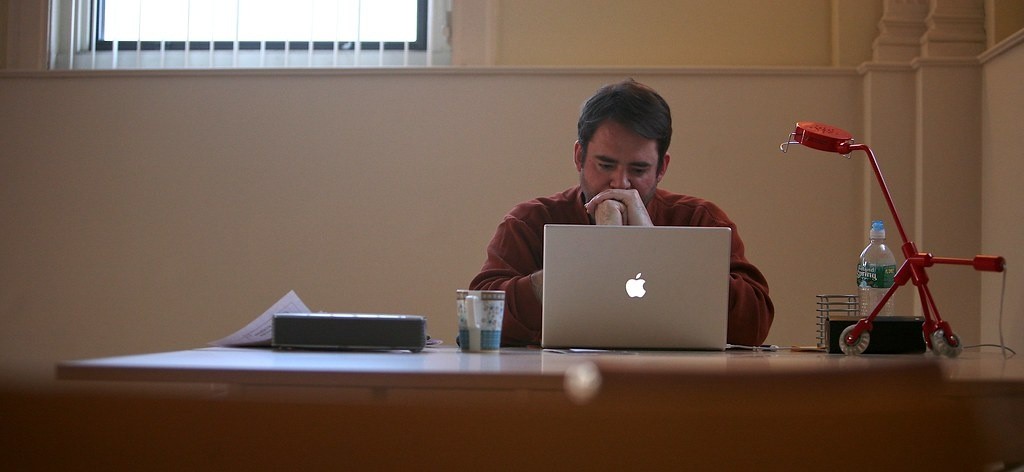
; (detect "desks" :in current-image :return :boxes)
[58,345,1024,472]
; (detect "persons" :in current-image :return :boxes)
[468,77,774,346]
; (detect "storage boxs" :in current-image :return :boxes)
[825,315,928,355]
[271,313,430,353]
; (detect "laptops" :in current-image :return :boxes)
[542,223,731,352]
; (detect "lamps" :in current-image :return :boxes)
[781,121,1008,357]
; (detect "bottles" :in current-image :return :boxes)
[856,221,897,318]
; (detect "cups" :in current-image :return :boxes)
[456,289,505,351]
